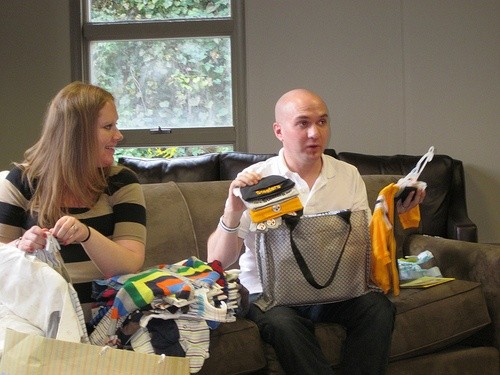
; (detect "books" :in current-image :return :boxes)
[400,275,456,288]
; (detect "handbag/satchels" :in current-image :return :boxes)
[252,209,386,312]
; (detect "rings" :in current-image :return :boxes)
[72,225,79,231]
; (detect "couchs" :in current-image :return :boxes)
[140,175,500,374]
[118,149,477,243]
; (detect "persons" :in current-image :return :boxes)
[0,81,148,335]
[207,87,426,375]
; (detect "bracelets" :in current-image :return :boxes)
[81,224,91,243]
[15,236,22,248]
[219,215,241,234]
